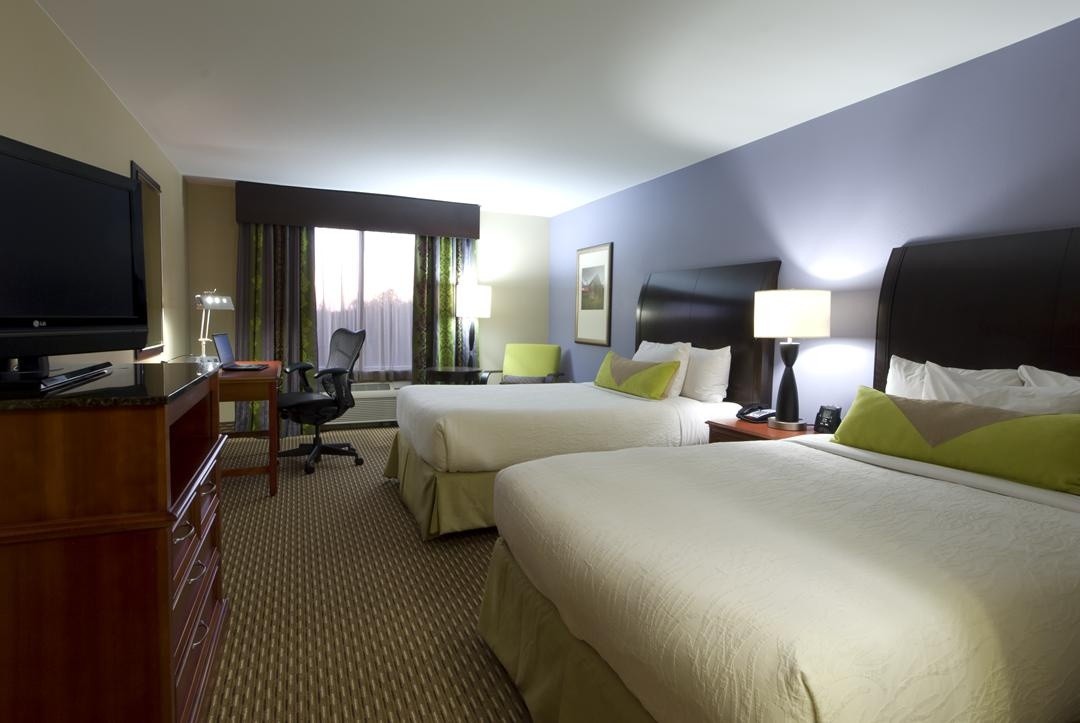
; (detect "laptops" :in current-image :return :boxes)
[212,332,269,372]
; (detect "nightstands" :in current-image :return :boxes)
[706,418,816,442]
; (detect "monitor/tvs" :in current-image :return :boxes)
[0,133,148,390]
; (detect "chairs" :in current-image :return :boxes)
[480,342,568,384]
[278,328,366,475]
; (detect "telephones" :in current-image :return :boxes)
[736,403,776,423]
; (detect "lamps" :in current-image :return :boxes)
[187,287,235,362]
[455,283,493,368]
[753,288,832,431]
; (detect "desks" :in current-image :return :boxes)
[425,366,482,385]
[219,360,283,497]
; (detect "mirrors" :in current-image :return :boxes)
[129,159,164,361]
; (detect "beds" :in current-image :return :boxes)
[479,228,1080,722]
[382,260,782,541]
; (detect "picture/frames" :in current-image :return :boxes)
[573,241,614,347]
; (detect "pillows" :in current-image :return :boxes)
[885,354,1023,399]
[829,385,1080,497]
[923,361,1080,413]
[594,351,681,400]
[632,340,692,399]
[674,342,732,403]
[1018,364,1080,388]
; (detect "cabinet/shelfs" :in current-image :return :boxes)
[0,372,231,723]
